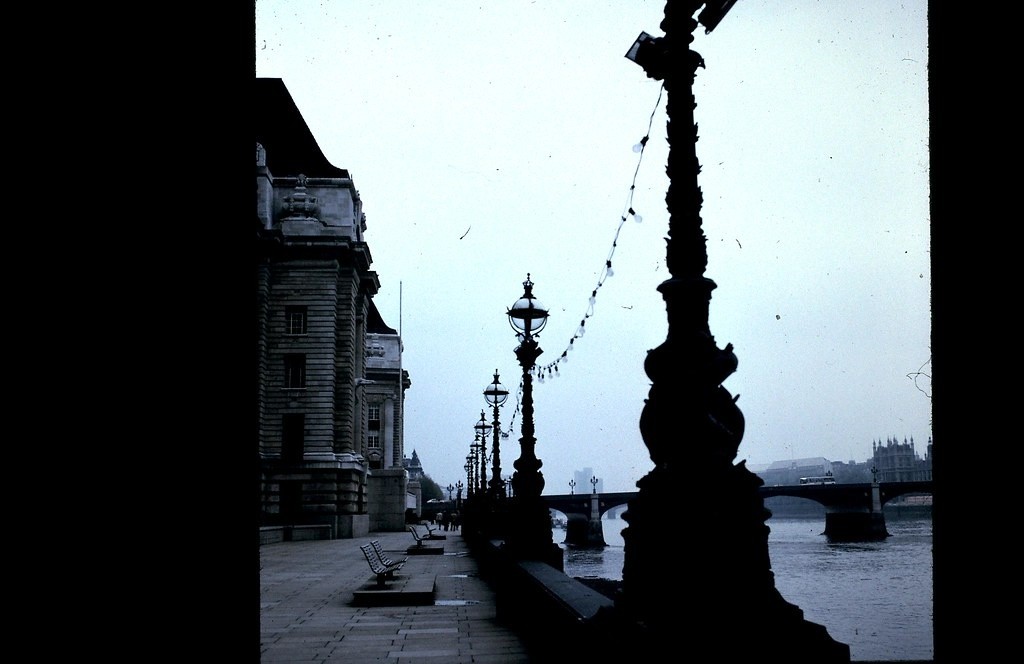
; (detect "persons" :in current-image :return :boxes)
[436,510,443,530]
[449,510,461,532]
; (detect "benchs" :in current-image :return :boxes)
[360,544,404,589]
[371,539,408,582]
[409,522,436,549]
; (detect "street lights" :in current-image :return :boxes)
[504,272,546,498]
[474,409,493,488]
[464,435,483,497]
[482,368,507,488]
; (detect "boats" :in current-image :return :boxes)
[562,516,607,551]
[824,510,891,543]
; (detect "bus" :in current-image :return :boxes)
[800,476,837,486]
